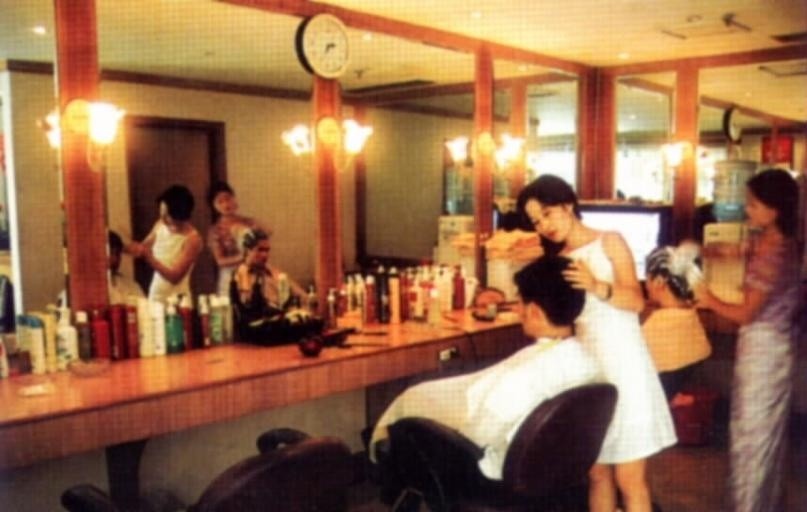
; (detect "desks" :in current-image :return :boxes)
[0,308,529,481]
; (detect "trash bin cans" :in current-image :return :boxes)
[669,385,719,445]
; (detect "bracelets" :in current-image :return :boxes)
[602,285,612,303]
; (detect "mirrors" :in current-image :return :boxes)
[617,77,673,203]
[695,98,807,219]
[0,2,80,333]
[485,49,586,226]
[79,2,331,301]
[524,80,578,197]
[356,84,526,267]
[330,29,485,307]
[694,58,807,222]
[599,75,678,205]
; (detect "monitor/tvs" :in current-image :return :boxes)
[575,200,672,292]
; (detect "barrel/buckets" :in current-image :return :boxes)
[710,159,756,222]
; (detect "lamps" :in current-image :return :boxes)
[64,98,125,175]
[280,127,314,177]
[318,116,375,173]
[482,132,523,175]
[445,137,474,184]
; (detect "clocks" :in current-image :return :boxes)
[726,106,742,144]
[299,13,352,77]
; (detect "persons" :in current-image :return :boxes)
[645,245,701,405]
[684,168,800,511]
[375,256,605,487]
[106,231,145,309]
[209,183,267,296]
[123,184,203,308]
[521,175,678,512]
[231,232,311,326]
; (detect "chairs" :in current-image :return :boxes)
[654,330,732,406]
[359,382,619,511]
[58,428,349,512]
